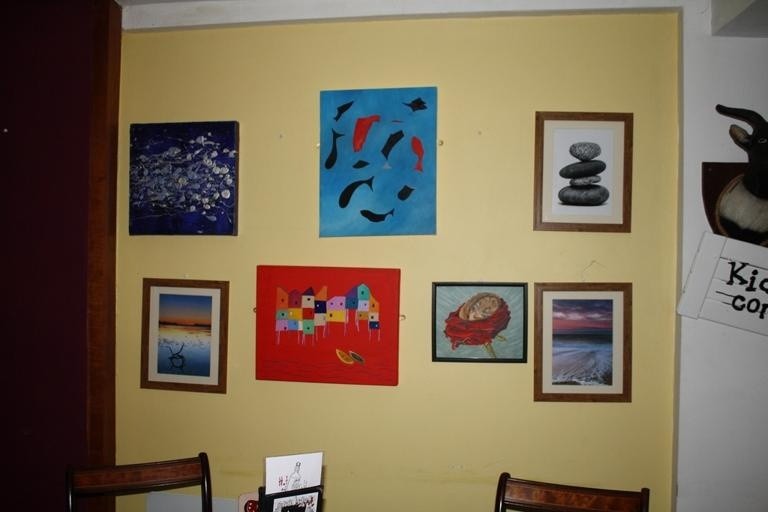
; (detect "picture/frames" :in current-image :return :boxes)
[533,281,633,402]
[431,281,528,364]
[141,278,229,393]
[532,111,633,232]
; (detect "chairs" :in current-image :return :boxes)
[494,472,650,512]
[67,453,212,512]
